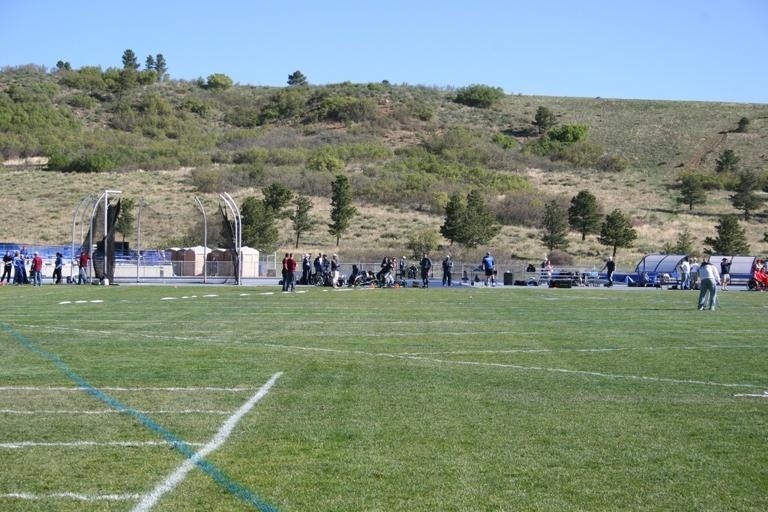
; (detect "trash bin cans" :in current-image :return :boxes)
[504,273,513,285]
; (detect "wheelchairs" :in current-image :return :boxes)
[748,275,766,291]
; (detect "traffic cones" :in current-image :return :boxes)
[394,283,400,288]
[369,283,376,288]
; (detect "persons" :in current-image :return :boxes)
[526,263,536,285]
[482,252,496,288]
[278,251,433,293]
[599,256,615,286]
[0,245,91,287]
[540,257,548,279]
[439,255,453,287]
[698,262,721,312]
[676,255,732,292]
[544,260,553,288]
[753,260,764,291]
